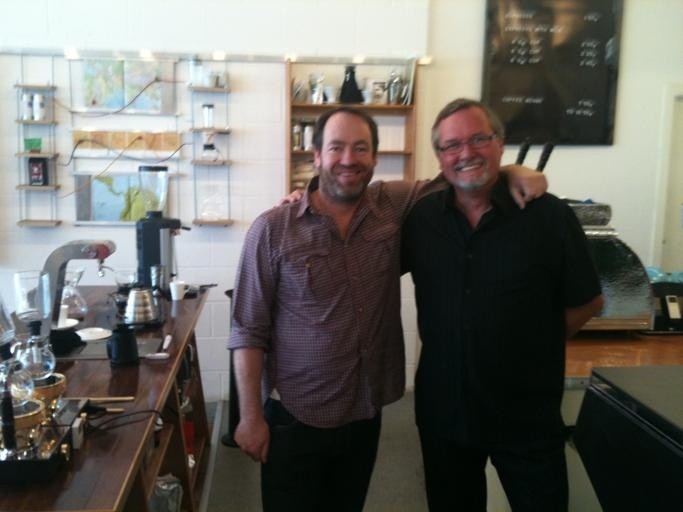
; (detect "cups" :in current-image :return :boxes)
[168,280,190,301]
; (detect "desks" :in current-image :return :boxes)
[0,286,210,512]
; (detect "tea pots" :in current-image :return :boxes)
[8,334,56,381]
[0,358,33,409]
[123,281,169,332]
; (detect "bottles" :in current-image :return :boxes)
[201,104,215,127]
[20,92,48,121]
[291,118,314,151]
[202,144,218,162]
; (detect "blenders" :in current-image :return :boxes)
[136,165,192,297]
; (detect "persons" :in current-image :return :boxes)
[276,96,605,511]
[230,108,552,511]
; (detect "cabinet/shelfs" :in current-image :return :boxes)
[0,48,430,227]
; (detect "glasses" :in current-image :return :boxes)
[435,131,499,156]
